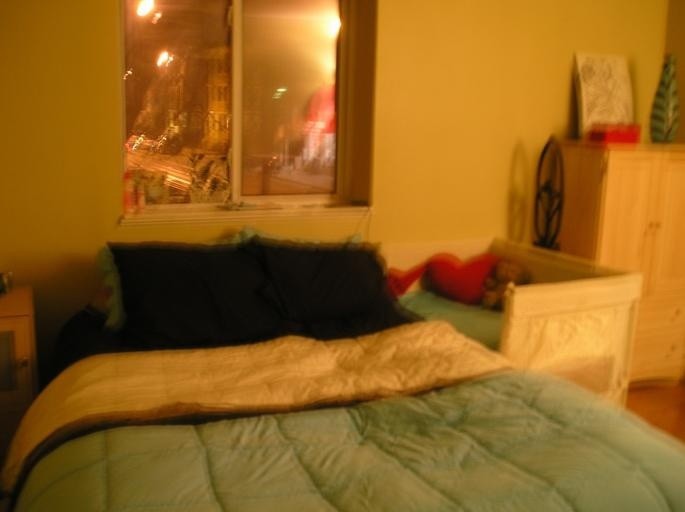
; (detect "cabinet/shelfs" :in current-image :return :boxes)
[558,140,685,391]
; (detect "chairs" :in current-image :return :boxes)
[393,234,645,408]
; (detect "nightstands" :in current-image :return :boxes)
[2,285,35,411]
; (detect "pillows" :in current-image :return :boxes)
[94,226,425,348]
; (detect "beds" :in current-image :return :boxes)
[0,337,685,511]
